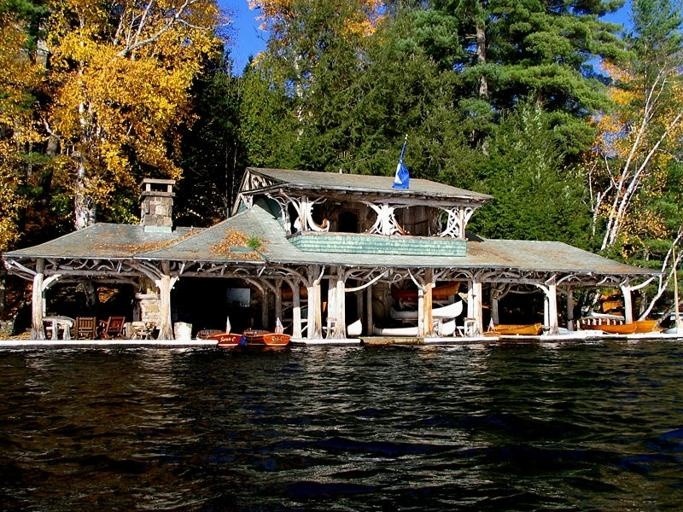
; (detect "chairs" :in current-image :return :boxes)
[97,316,125,340]
[73,317,96,339]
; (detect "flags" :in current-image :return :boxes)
[392,144,409,189]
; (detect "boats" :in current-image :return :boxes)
[582,323,637,334]
[496,322,540,335]
[393,281,461,301]
[636,320,658,331]
[196,326,291,347]
[372,318,455,336]
[389,300,463,320]
